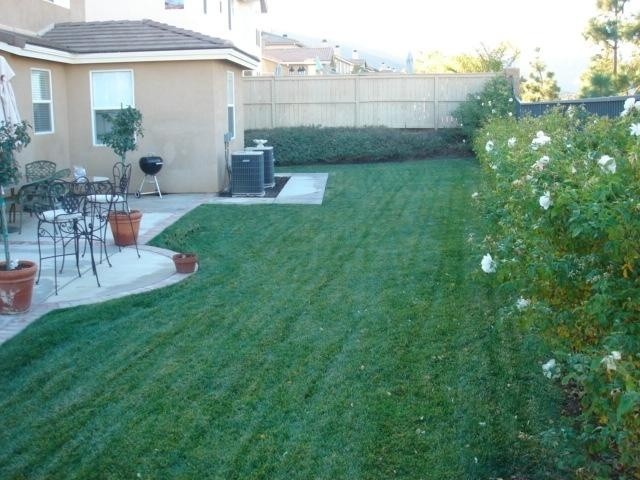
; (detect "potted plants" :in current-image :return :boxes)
[0,120,42,315]
[106,105,142,246]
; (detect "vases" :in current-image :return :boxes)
[173,253,197,273]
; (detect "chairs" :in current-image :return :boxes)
[16,160,141,295]
